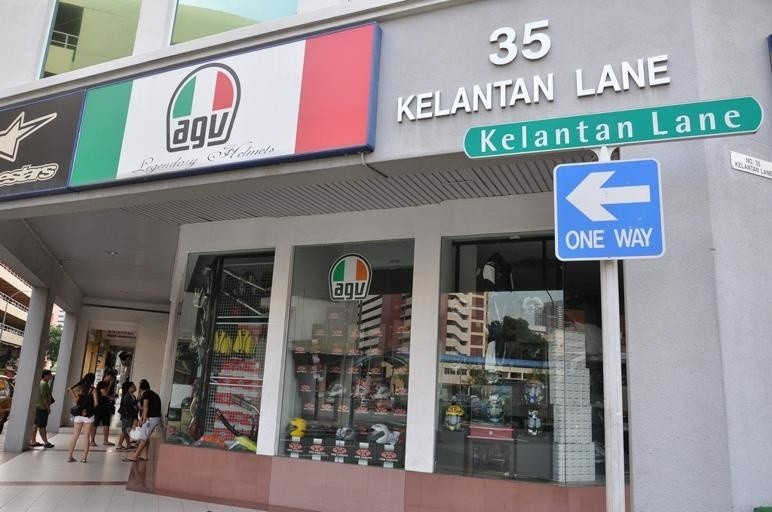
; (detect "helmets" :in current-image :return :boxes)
[445,406,465,432]
[372,381,391,399]
[335,425,357,441]
[485,393,506,423]
[352,382,371,396]
[524,410,546,438]
[521,379,546,408]
[328,382,347,396]
[288,418,306,436]
[369,423,393,444]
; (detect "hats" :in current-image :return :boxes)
[4,366,15,372]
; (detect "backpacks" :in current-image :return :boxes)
[213,329,255,354]
[240,272,258,296]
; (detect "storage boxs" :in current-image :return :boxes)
[213,359,262,442]
[285,307,409,469]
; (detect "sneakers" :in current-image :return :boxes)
[44,443,54,448]
[31,442,44,446]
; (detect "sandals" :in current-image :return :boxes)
[67,457,76,462]
[80,458,86,463]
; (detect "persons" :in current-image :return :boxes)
[31,369,56,448]
[89,368,163,462]
[67,372,99,463]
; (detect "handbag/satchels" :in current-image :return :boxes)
[50,395,55,404]
[118,406,133,418]
[70,404,84,416]
[126,426,148,442]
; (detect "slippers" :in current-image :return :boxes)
[89,443,97,446]
[103,442,136,450]
[122,456,148,462]
